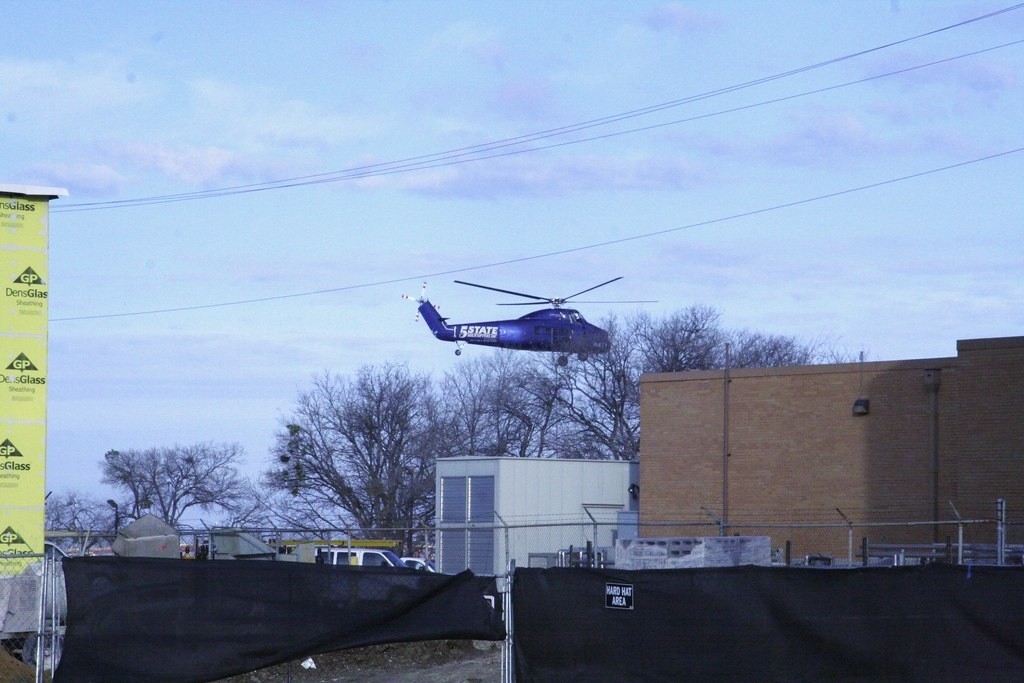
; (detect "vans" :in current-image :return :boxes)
[314,549,407,569]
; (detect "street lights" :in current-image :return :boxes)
[107,499,119,535]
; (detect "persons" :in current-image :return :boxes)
[183,539,209,560]
[314,548,324,564]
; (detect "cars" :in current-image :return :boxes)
[398,557,435,573]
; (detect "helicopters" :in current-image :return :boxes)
[401,275,624,366]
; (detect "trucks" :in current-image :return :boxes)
[22,540,70,671]
